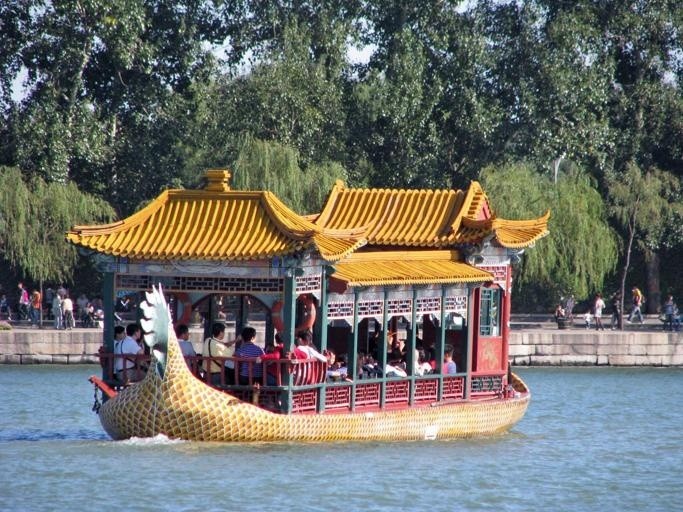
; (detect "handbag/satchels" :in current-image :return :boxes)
[70,315,76,328]
[133,357,149,371]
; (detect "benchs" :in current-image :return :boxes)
[95,352,329,388]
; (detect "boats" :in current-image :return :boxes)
[65,168,553,444]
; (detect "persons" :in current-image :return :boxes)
[110,320,456,390]
[0,282,251,329]
[553,283,682,331]
